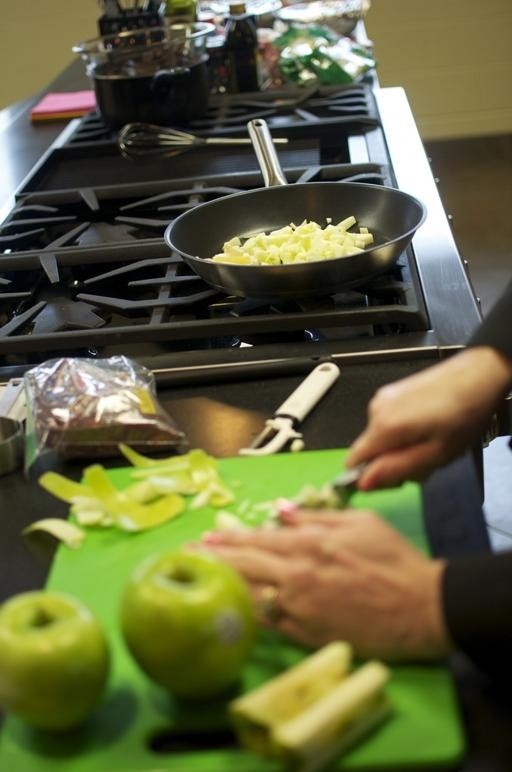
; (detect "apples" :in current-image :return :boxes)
[0,589,112,734]
[118,546,257,700]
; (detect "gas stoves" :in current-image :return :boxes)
[1,87,483,378]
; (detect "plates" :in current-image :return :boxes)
[71,22,217,72]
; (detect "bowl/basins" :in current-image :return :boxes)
[275,3,371,36]
[92,54,210,133]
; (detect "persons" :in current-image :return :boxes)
[182,277,512,665]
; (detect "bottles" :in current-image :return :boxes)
[222,2,261,93]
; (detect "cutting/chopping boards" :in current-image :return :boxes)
[0,447,462,768]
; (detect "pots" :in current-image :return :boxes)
[162,115,426,296]
